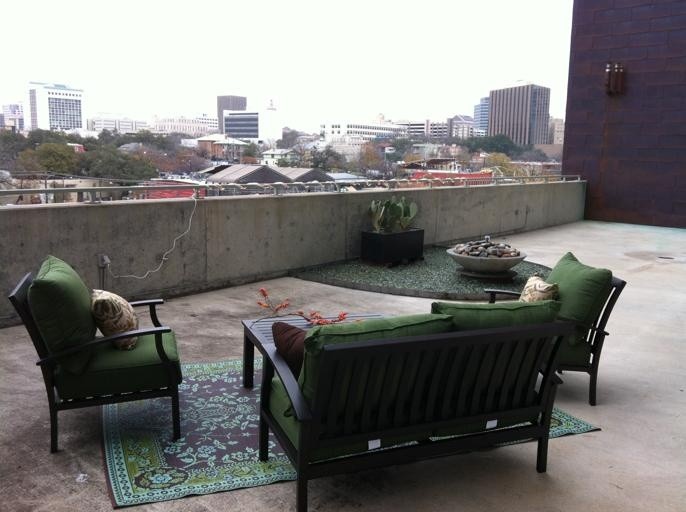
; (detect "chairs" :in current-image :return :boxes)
[484,275,626,406]
[8,272,183,453]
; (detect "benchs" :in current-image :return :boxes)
[259,320,576,512]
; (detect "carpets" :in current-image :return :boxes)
[101,357,601,507]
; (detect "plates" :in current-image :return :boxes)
[445,246,527,272]
[455,266,518,280]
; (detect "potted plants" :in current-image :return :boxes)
[361,196,425,267]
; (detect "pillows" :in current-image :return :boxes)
[27,254,97,384]
[90,287,139,352]
[519,271,559,303]
[272,321,307,371]
[431,300,562,331]
[283,313,454,417]
[544,252,612,348]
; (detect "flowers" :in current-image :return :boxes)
[250,288,360,326]
